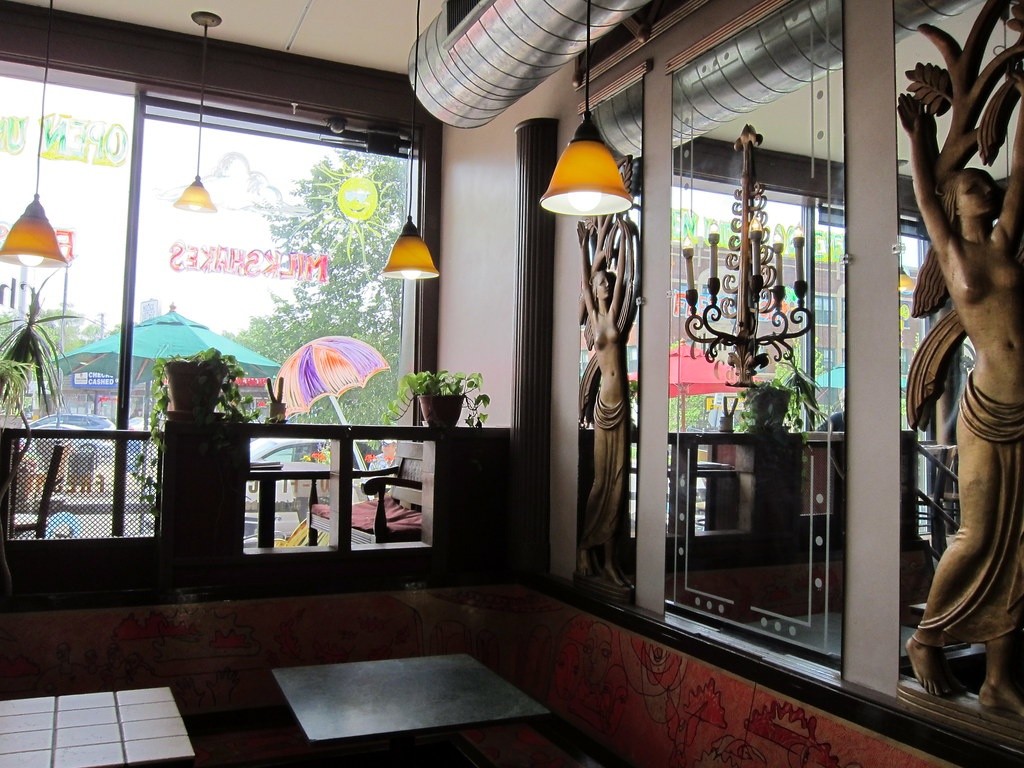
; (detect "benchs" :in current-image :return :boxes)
[0,584,963,768]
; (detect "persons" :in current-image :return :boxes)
[575,218,630,583]
[369,440,397,491]
[895,62,1024,718]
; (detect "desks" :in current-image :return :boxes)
[0,686,196,768]
[667,466,739,531]
[272,652,553,768]
[247,461,331,549]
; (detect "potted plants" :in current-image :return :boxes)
[131,347,284,517]
[737,377,809,445]
[720,396,738,431]
[267,374,287,417]
[379,368,491,428]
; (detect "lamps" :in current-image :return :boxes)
[539,0,634,217]
[0,0,68,268]
[380,0,439,279]
[897,225,916,291]
[173,10,223,214]
[683,123,813,387]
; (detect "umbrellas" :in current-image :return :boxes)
[52,305,281,536]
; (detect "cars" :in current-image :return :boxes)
[129,417,166,431]
[19,413,115,467]
[245,438,381,510]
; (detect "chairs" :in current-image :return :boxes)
[918,444,958,554]
[307,441,423,546]
[10,439,73,538]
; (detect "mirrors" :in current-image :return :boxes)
[662,0,850,668]
[573,59,651,607]
[891,0,1024,716]
